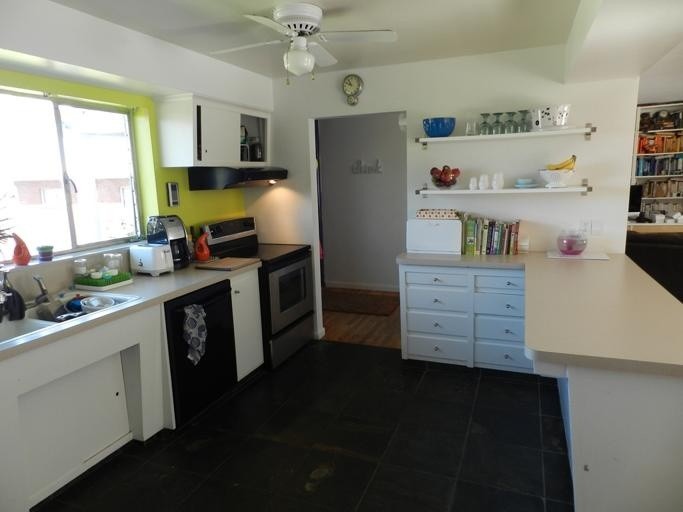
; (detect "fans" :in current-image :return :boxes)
[204,1,398,68]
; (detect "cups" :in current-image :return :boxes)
[72,253,122,280]
[517,178,534,184]
[37,246,52,262]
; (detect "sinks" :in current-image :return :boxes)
[0,312,59,344]
[23,289,143,324]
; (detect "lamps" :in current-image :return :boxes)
[279,35,316,88]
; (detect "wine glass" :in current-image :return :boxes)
[463,108,531,135]
[537,170,574,189]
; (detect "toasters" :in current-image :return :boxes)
[130,243,175,278]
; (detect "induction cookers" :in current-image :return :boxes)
[223,244,309,264]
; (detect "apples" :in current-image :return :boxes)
[431,166,460,186]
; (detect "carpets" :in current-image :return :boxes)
[30,336,575,510]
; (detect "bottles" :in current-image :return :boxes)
[469,173,504,190]
[185,235,195,261]
[240,124,263,163]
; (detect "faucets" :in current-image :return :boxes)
[24,276,50,311]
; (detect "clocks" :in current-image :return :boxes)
[341,72,366,107]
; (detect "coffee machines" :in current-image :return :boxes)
[145,214,189,270]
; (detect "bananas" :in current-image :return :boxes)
[545,155,576,170]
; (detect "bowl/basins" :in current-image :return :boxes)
[532,104,572,126]
[420,119,456,137]
[431,176,457,191]
[557,230,587,256]
[53,293,114,320]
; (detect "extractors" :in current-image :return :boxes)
[188,167,290,192]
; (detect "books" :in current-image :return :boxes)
[633,110,682,221]
[461,212,520,256]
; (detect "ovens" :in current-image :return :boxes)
[255,253,313,358]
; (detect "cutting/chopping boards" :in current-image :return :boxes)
[195,257,260,272]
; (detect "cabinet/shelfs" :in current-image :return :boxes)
[413,128,598,193]
[228,266,263,381]
[630,101,682,237]
[396,249,532,373]
[12,343,133,504]
[158,95,273,168]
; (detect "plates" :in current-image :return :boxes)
[514,184,538,189]
[535,126,568,132]
